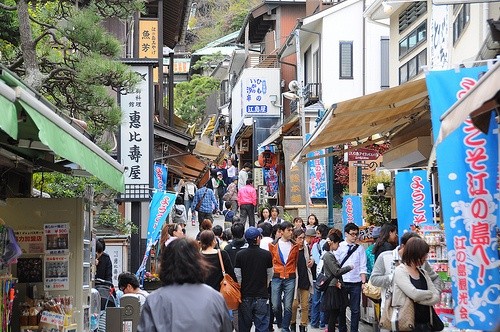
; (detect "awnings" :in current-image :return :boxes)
[257,59,500,182]
[0,64,126,194]
[167,136,223,183]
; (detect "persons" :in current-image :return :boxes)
[94,239,112,283]
[117,158,443,332]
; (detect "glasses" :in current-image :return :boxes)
[347,232,359,237]
[297,237,305,240]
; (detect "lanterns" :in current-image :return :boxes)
[257,150,277,171]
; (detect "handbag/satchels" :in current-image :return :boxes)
[188,194,194,201]
[314,273,332,291]
[195,200,203,211]
[98,295,124,332]
[220,274,242,310]
[221,191,231,201]
[378,265,415,331]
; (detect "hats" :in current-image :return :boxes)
[245,226,263,241]
[305,229,317,236]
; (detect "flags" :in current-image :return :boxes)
[258,143,278,196]
[134,164,176,278]
[304,132,327,199]
[424,63,500,330]
[394,169,436,265]
[341,195,363,240]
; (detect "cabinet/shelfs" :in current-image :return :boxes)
[418,227,454,316]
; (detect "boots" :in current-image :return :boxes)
[299,325,305,332]
[290,322,296,332]
